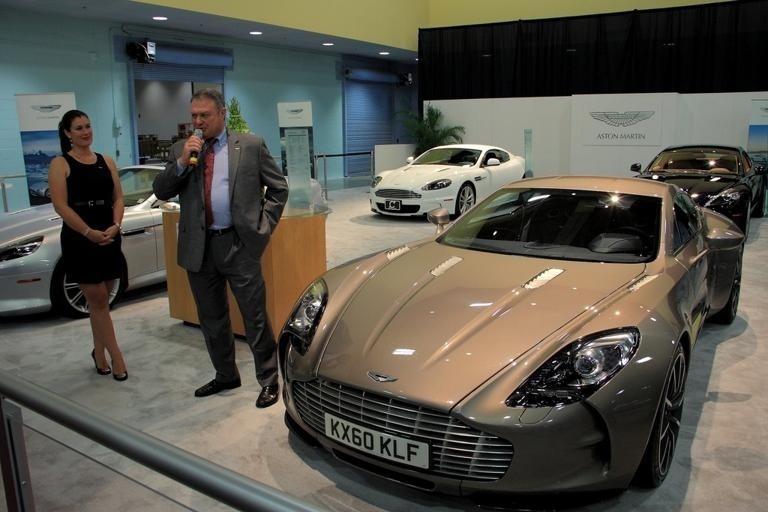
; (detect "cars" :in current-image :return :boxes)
[30,179,51,203]
[0,165,328,319]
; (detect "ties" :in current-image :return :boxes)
[254,380,280,409]
[201,142,216,228]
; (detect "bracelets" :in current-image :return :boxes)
[111,222,122,231]
[83,226,92,236]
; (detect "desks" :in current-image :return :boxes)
[160,210,328,341]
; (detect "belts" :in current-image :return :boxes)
[204,224,235,237]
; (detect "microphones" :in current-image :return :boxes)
[190,129,204,171]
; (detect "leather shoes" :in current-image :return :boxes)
[110,356,129,382]
[193,373,242,397]
[91,347,112,375]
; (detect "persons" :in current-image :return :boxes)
[48,109,129,381]
[151,88,291,409]
